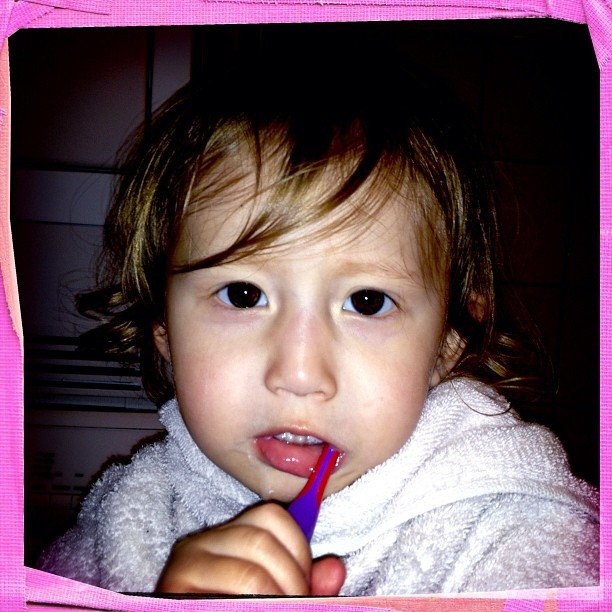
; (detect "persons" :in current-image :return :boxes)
[38,77,599,596]
